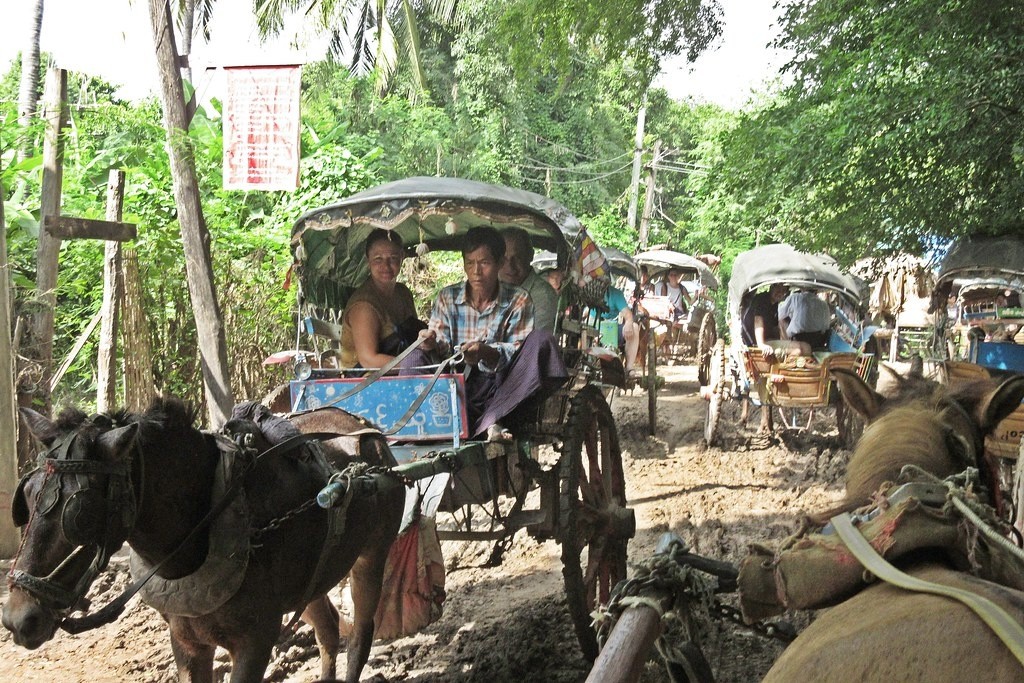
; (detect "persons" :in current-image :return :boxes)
[994,293,1007,308]
[778,287,832,350]
[489,228,558,338]
[339,228,427,377]
[396,227,570,444]
[624,266,655,297]
[545,267,578,350]
[581,284,639,376]
[743,283,790,358]
[661,268,699,330]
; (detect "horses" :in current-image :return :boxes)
[759,354,1024,683]
[1,393,407,683]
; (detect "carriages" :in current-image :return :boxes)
[2,176,637,683]
[529,235,1023,683]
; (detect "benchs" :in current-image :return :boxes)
[306,281,1024,458]
